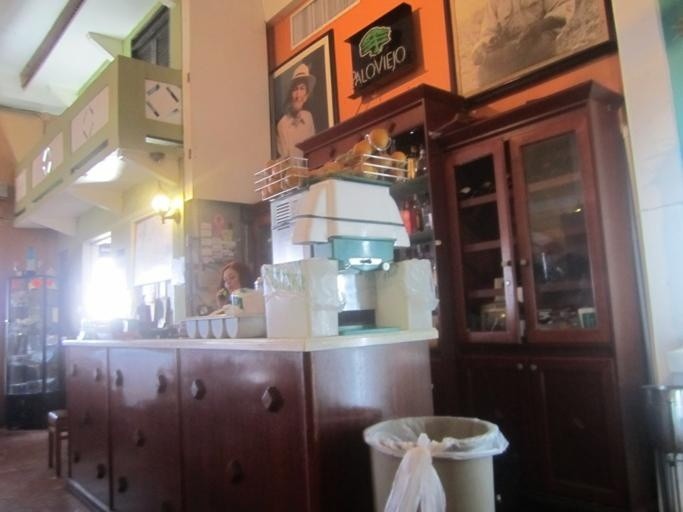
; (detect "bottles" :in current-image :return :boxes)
[534,246,554,284]
[400,144,434,237]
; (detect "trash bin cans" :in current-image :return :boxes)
[363,416,499,512]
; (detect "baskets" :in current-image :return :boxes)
[350,153,414,182]
[252,157,309,202]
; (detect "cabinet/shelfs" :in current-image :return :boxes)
[292,78,456,421]
[1,273,63,426]
[446,79,655,512]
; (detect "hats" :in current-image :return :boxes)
[287,64,316,99]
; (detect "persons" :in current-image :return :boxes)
[216,260,252,308]
[277,62,318,159]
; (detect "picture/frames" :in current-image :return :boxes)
[269,30,340,161]
[442,1,620,106]
